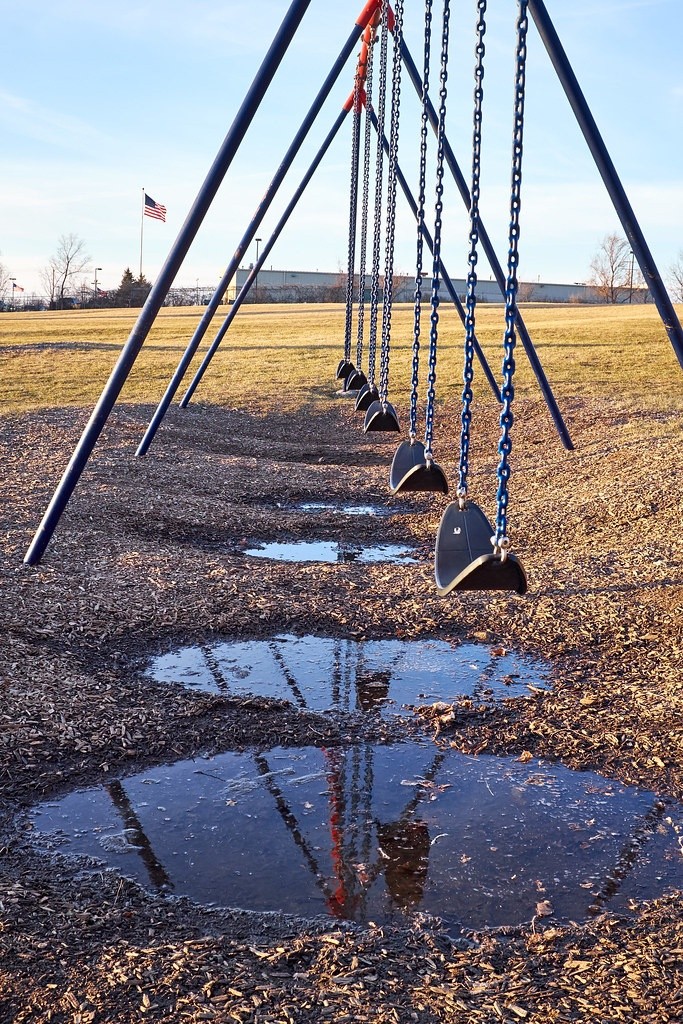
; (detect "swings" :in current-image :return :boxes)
[389,0,450,494]
[332,0,403,432]
[434,0,533,594]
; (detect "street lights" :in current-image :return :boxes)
[95,268,102,292]
[630,251,634,303]
[255,238,262,305]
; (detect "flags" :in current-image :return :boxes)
[97,287,107,298]
[13,283,24,293]
[144,194,167,222]
